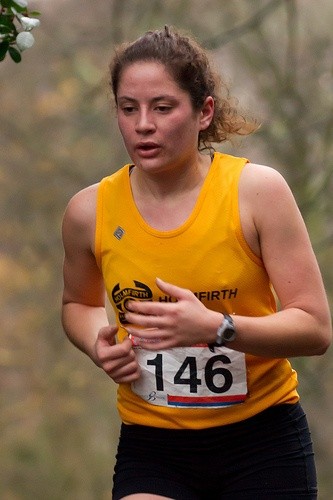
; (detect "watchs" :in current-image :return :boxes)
[207,309,237,353]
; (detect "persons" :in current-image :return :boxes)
[62,22,333,500]
